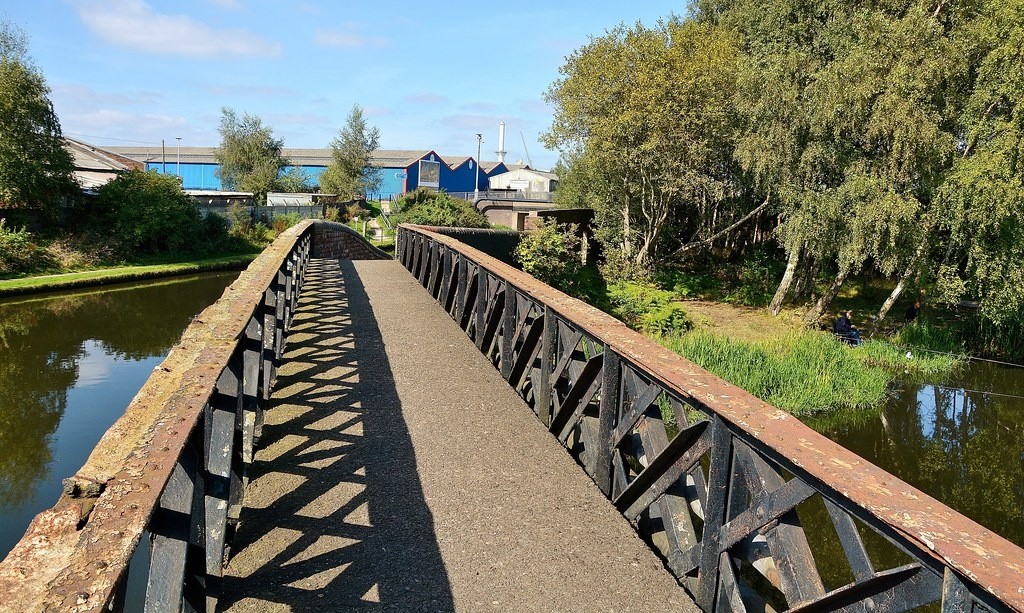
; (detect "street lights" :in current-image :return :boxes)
[474,134,485,201]
[176,137,182,177]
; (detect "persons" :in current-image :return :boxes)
[836,309,861,346]
[905,300,921,322]
